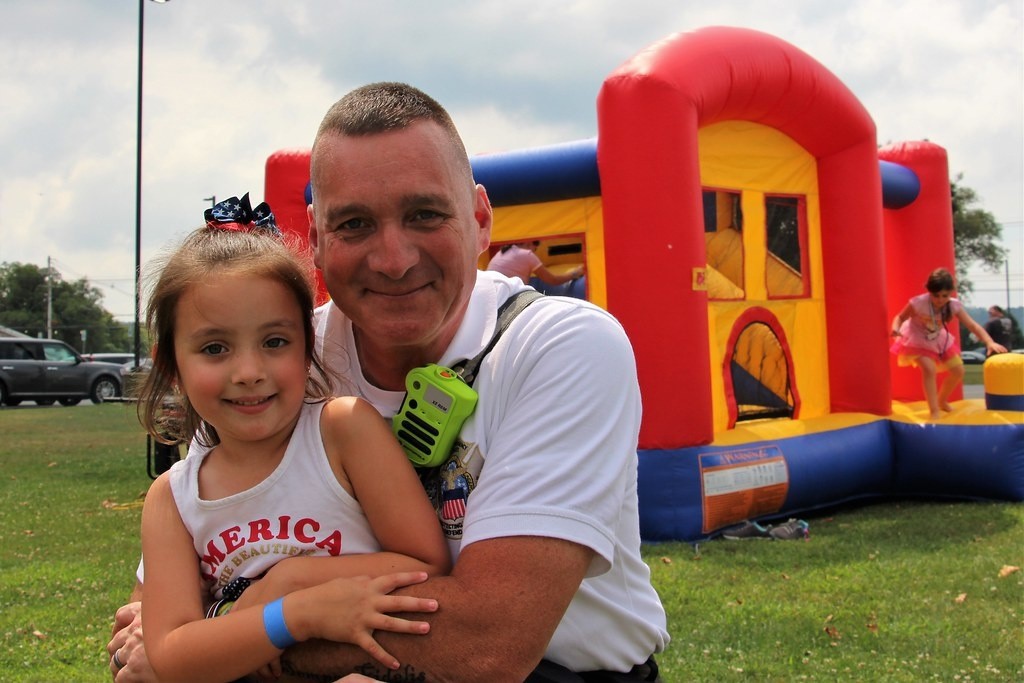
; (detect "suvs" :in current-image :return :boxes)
[0,338,126,407]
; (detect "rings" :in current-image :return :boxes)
[114,649,123,669]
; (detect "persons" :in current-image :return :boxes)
[107,84,669,683]
[137,228,448,683]
[984,306,1013,357]
[890,268,1008,416]
[488,239,584,286]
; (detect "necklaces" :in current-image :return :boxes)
[930,301,948,362]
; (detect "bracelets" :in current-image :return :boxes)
[263,597,301,650]
[205,599,234,619]
